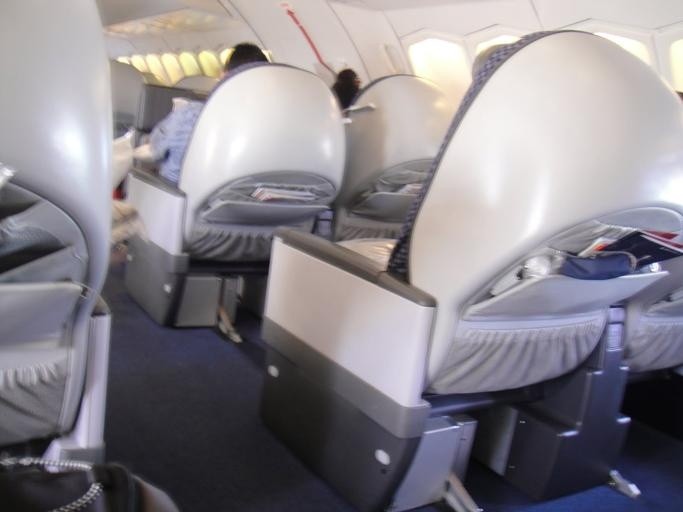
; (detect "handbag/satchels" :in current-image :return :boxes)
[2,458,140,511]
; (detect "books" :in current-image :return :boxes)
[575,228,683,270]
[397,182,422,196]
[250,180,319,208]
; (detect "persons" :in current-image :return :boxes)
[330,71,365,110]
[141,41,269,185]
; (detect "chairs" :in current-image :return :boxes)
[106,52,450,331]
[0,1,117,506]
[253,30,680,512]
